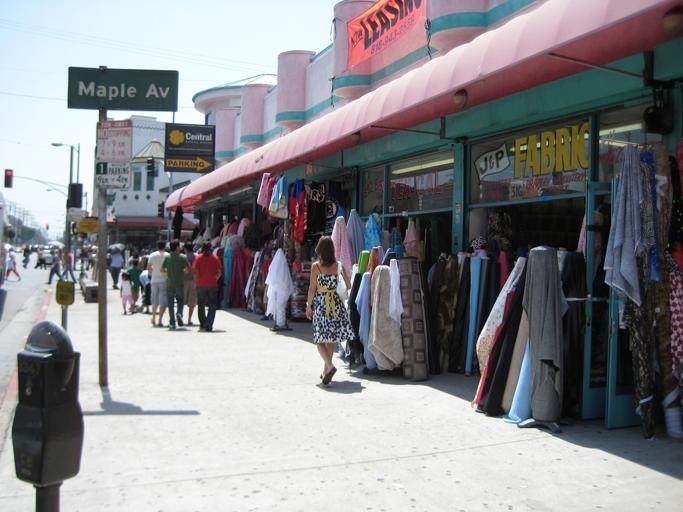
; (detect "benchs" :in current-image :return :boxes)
[80,278,99,303]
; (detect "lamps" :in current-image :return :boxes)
[350,131,362,145]
[452,88,468,110]
[662,6,682,34]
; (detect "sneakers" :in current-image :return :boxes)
[151,313,212,333]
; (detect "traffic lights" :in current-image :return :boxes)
[5,169,12,187]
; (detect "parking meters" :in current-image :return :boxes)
[11,321,86,511]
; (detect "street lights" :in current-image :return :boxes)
[52,142,79,271]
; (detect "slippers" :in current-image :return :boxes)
[322,367,337,385]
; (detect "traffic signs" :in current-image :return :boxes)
[164,122,216,174]
[67,66,179,112]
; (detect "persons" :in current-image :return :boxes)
[148,240,171,326]
[35,233,160,315]
[160,238,191,329]
[23,245,30,268]
[192,243,223,331]
[176,241,199,324]
[306,302,312,305]
[6,248,22,281]
[305,235,352,385]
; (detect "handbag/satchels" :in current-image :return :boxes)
[336,261,349,301]
